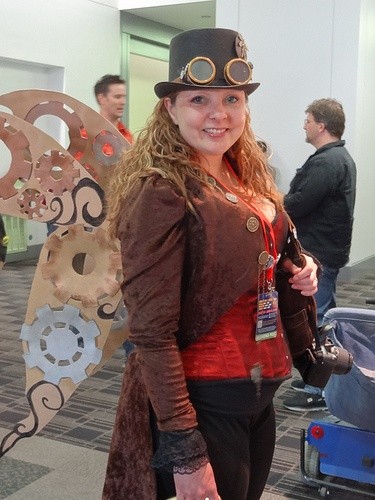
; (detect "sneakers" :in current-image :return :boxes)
[282,390,328,411]
[291,380,306,390]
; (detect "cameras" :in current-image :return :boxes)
[294,325,353,388]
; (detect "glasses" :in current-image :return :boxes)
[170,56,253,86]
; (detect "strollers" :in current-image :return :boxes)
[299,299,375,497]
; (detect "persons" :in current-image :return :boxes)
[70,75,136,358]
[103,28,325,500]
[275,97,357,413]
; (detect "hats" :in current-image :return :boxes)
[154,28,260,99]
